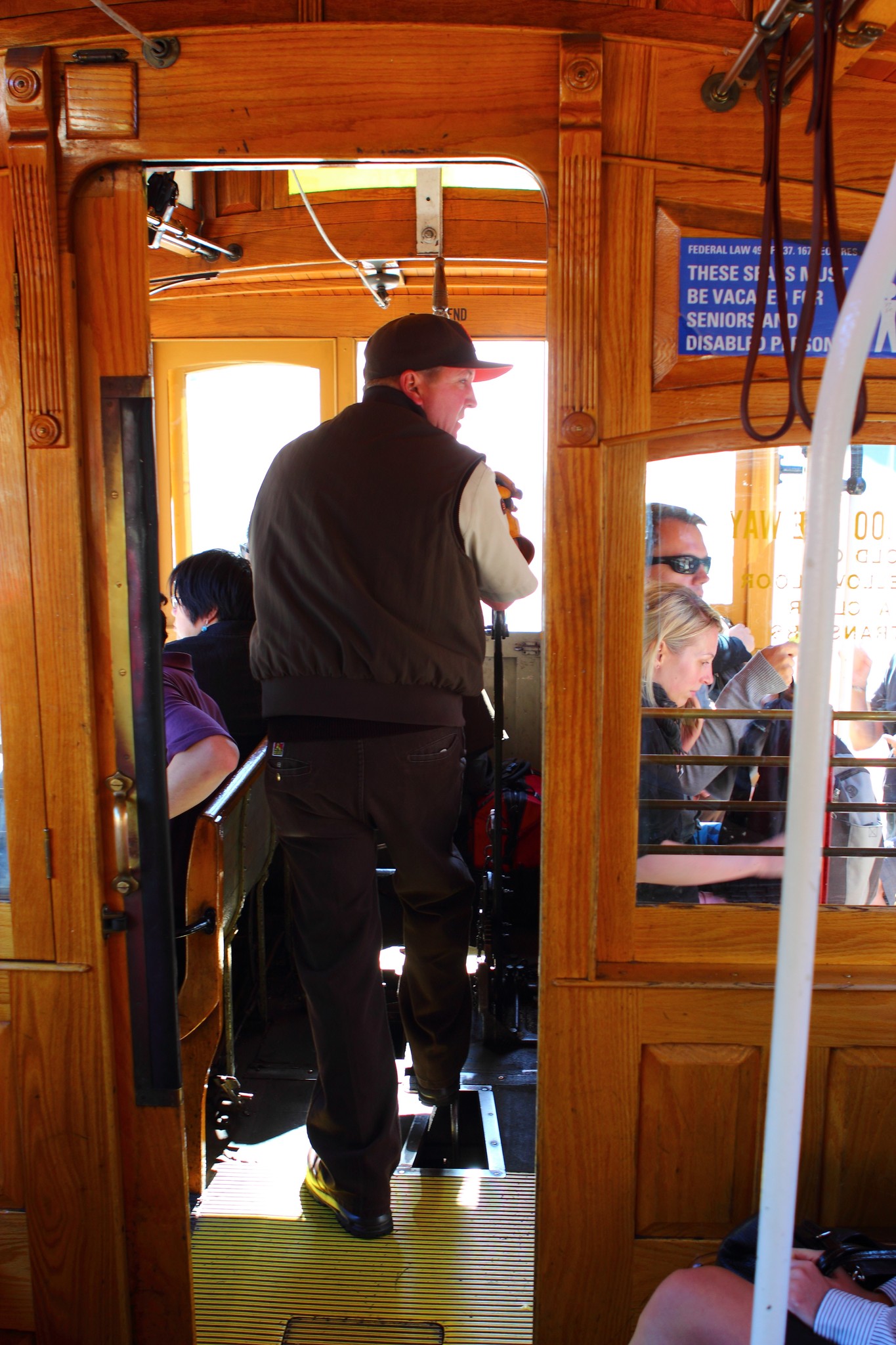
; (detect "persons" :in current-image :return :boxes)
[629,1247,896,1345]
[693,605,896,905]
[170,548,267,762]
[249,313,537,1240]
[153,592,239,992]
[633,582,786,904]
[641,502,796,844]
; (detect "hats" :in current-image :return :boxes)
[363,312,513,382]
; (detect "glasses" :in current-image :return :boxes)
[171,596,183,608]
[647,555,711,574]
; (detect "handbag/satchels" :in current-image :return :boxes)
[714,1215,896,1292]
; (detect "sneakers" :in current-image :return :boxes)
[417,1085,459,1105]
[305,1146,394,1240]
